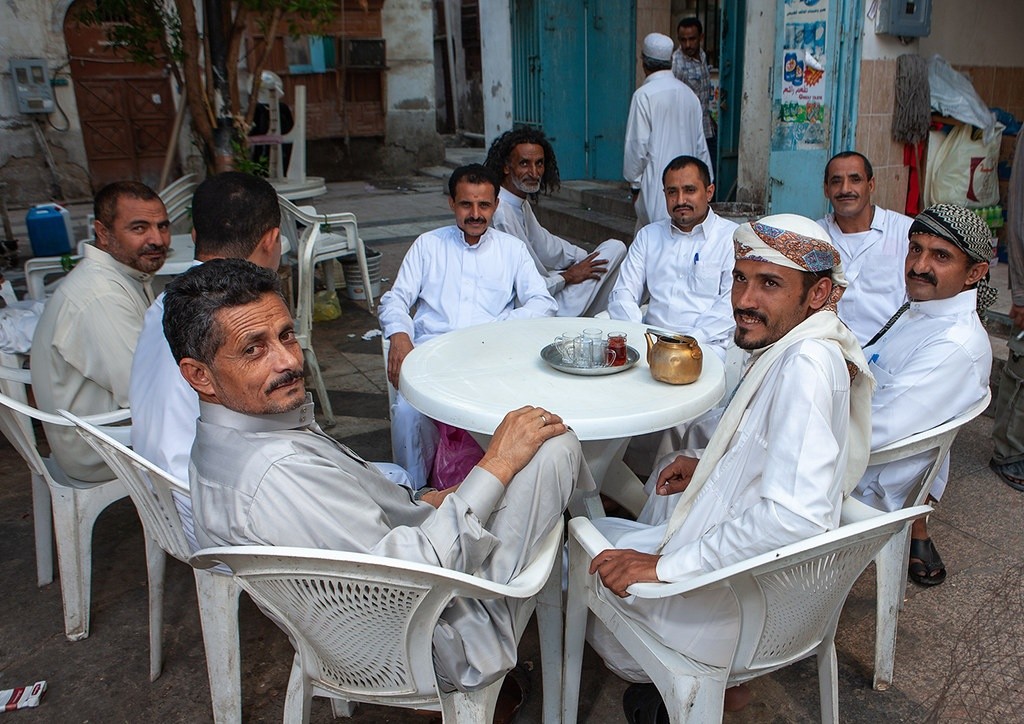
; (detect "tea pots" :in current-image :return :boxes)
[644,328,703,384]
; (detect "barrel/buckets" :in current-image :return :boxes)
[25,202,76,257]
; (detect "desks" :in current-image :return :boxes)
[155,233,291,276]
[216,86,327,206]
[397,316,726,521]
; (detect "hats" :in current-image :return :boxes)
[642,33,676,61]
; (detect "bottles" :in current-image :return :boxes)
[972,205,1002,221]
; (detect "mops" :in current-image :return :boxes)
[889,52,931,214]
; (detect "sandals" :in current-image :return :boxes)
[908,536,947,587]
[988,455,1024,491]
[493,663,531,724]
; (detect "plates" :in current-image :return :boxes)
[540,341,641,376]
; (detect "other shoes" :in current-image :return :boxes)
[622,682,671,724]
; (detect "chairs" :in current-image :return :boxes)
[0,281,133,643]
[56,409,565,724]
[561,495,935,724]
[25,172,198,301]
[277,193,374,425]
[867,386,992,692]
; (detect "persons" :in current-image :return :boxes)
[483,126,627,319]
[989,116,1024,493]
[31,180,171,483]
[129,170,282,575]
[671,17,716,166]
[623,32,714,240]
[607,153,741,468]
[377,162,558,490]
[161,256,582,692]
[559,213,875,724]
[680,202,999,514]
[814,151,950,586]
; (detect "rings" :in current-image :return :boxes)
[540,415,548,425]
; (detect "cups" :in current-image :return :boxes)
[554,328,627,368]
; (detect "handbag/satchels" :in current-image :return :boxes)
[923,113,1006,209]
